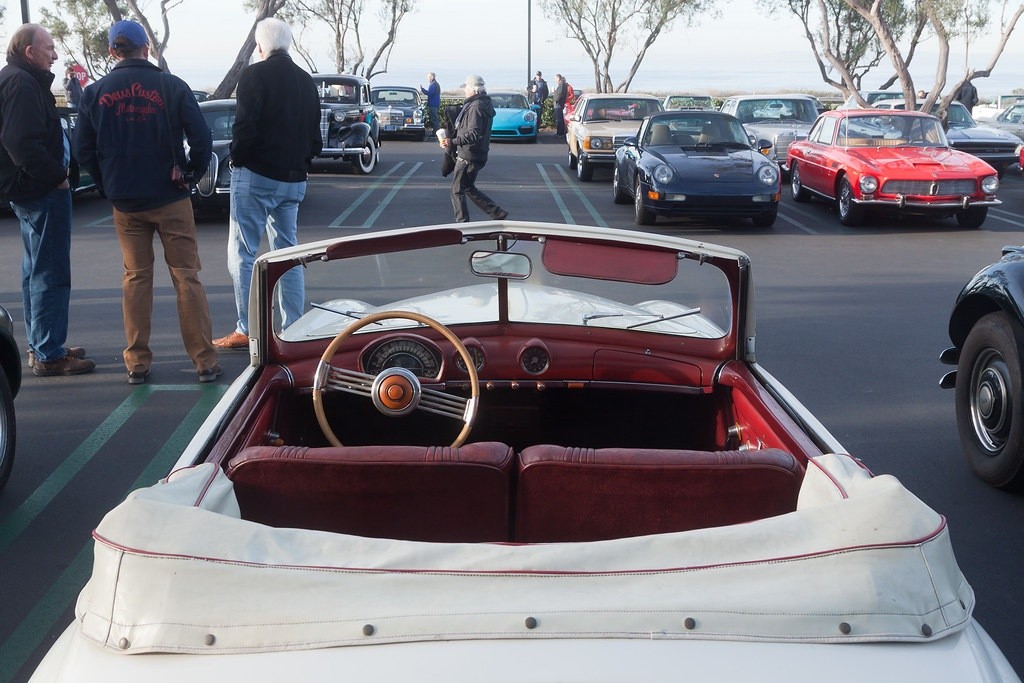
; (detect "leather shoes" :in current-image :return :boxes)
[212,331,249,351]
[128,368,151,383]
[199,364,223,382]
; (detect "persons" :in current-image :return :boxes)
[0,23,96,376]
[62,67,83,128]
[552,74,574,138]
[212,17,323,350]
[72,21,224,384]
[918,90,941,100]
[441,74,509,223]
[954,74,979,117]
[526,71,549,133]
[420,73,440,139]
[629,104,639,119]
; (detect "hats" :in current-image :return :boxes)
[460,75,485,88]
[536,71,541,76]
[109,20,149,50]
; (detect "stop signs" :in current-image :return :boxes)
[65,65,88,87]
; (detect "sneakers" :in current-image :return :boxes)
[33,356,96,376]
[28,347,85,368]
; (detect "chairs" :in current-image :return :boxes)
[491,99,522,107]
[591,106,606,119]
[1008,113,1022,122]
[649,122,723,147]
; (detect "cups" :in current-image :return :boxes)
[436,128,448,148]
[533,85,537,91]
[551,86,555,93]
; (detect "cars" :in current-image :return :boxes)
[28,219,1020,683]
[565,93,677,182]
[488,90,541,143]
[191,90,208,102]
[0,107,97,215]
[662,94,720,135]
[938,245,1024,495]
[718,94,821,165]
[752,93,829,120]
[835,90,904,110]
[573,90,583,100]
[309,73,382,176]
[612,110,782,228]
[180,97,231,220]
[971,94,1024,123]
[839,98,1024,181]
[369,86,426,142]
[977,101,1024,143]
[781,107,1003,230]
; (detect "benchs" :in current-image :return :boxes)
[840,138,908,146]
[224,439,805,543]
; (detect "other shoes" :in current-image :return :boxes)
[494,209,509,220]
